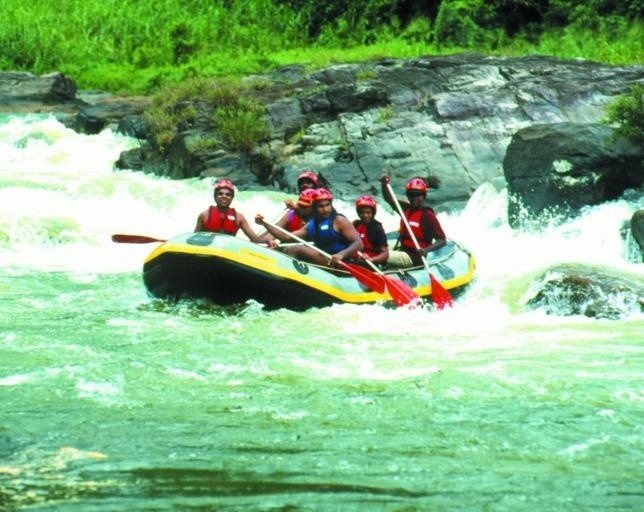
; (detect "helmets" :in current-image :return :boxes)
[298,171,377,209]
[406,179,428,192]
[214,179,234,193]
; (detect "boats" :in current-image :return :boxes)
[143,232,477,308]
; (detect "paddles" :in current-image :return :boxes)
[261,219,385,293]
[357,250,421,309]
[385,177,453,311]
[112,235,314,249]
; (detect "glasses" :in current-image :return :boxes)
[219,192,232,196]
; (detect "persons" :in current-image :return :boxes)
[258,191,317,245]
[350,196,390,269]
[379,175,444,269]
[190,176,277,250]
[255,188,364,269]
[298,171,320,189]
